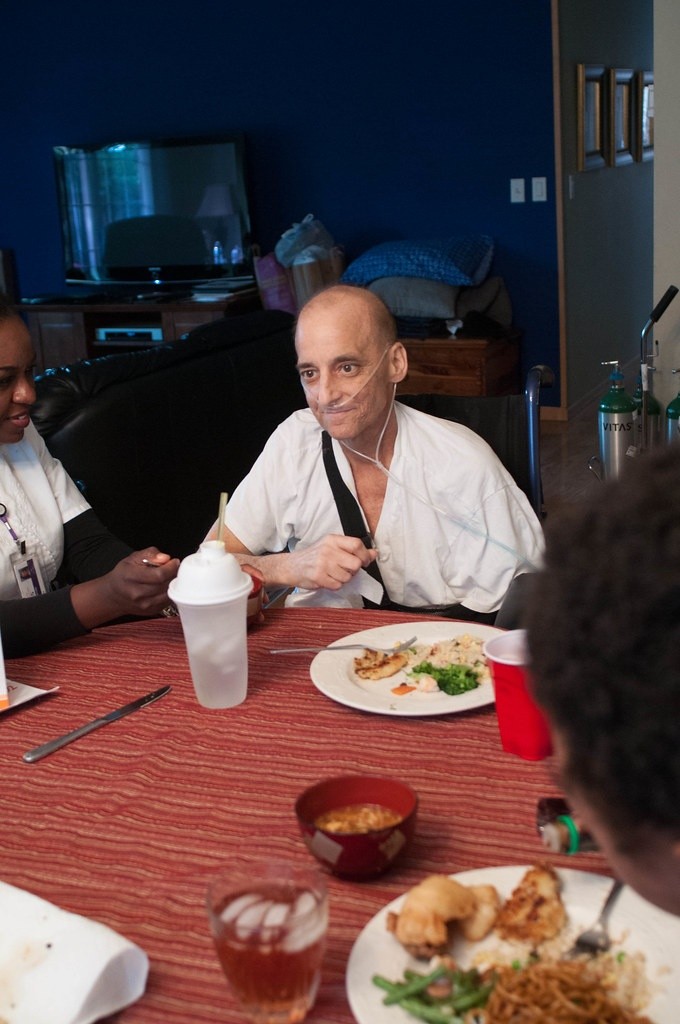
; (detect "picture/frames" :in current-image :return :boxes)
[577,63,654,172]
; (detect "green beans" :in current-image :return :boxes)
[373,965,527,1024]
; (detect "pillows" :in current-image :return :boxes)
[341,231,496,319]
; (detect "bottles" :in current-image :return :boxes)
[665,369,680,448]
[213,240,242,264]
[246,575,262,629]
[632,369,663,455]
[598,361,637,486]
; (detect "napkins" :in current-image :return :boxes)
[0,880,147,1024]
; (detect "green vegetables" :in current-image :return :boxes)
[407,661,480,695]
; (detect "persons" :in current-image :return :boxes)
[205,284,544,614]
[527,436,680,919]
[0,298,181,658]
[20,567,31,579]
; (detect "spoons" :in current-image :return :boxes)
[575,880,624,957]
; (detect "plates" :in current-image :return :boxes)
[309,621,506,716]
[346,865,680,1024]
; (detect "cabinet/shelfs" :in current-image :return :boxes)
[10,289,256,376]
[394,333,522,399]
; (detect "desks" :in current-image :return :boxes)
[0,605,625,1024]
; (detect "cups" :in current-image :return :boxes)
[482,628,552,761]
[167,541,254,709]
[210,862,328,1024]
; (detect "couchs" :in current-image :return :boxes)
[28,306,310,563]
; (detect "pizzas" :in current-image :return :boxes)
[494,866,569,953]
[352,647,406,680]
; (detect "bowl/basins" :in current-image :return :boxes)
[295,777,418,882]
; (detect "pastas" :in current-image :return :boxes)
[462,957,655,1024]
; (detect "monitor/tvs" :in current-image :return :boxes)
[52,127,274,303]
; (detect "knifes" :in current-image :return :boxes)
[22,684,172,762]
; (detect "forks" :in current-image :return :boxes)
[270,636,416,653]
